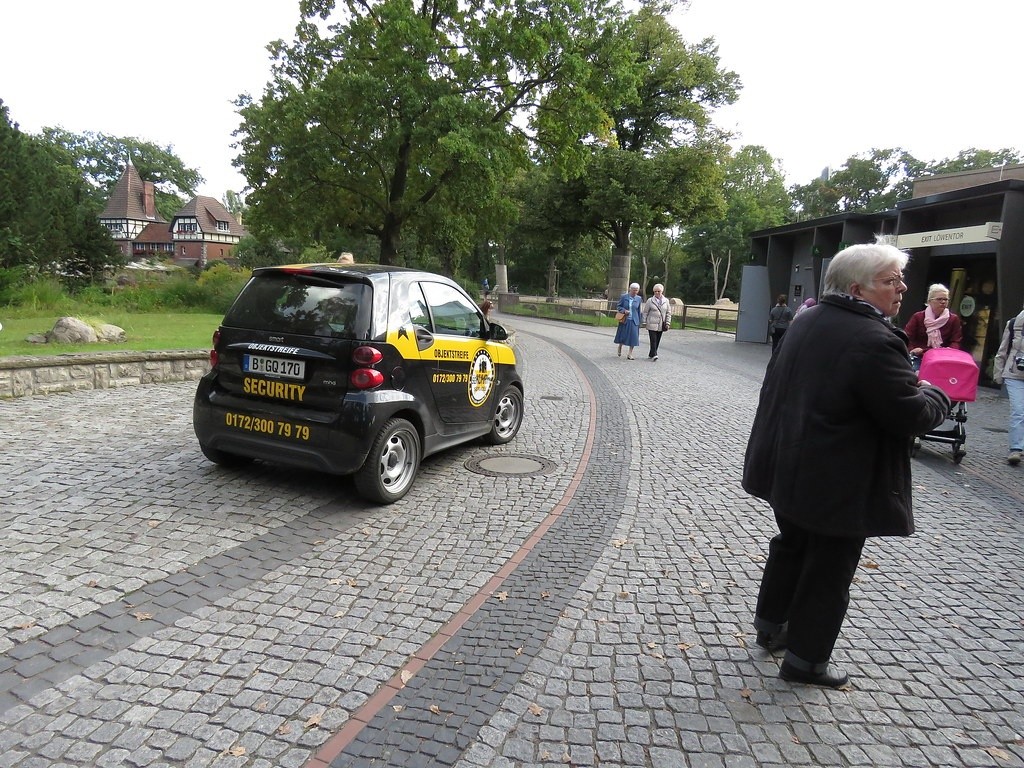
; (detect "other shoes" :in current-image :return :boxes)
[627,355,635,359]
[1008,452,1022,462]
[652,355,659,361]
[648,355,651,358]
[617,348,621,356]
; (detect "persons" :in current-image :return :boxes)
[768,295,793,355]
[483,278,490,303]
[614,283,641,360]
[742,233,951,686]
[904,283,963,371]
[994,309,1024,465]
[478,300,494,321]
[642,284,671,362]
[793,298,816,320]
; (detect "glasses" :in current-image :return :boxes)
[929,297,950,304]
[873,274,907,288]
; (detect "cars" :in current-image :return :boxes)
[193,263,524,504]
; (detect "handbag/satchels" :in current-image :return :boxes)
[769,323,776,335]
[615,313,626,322]
[662,322,668,331]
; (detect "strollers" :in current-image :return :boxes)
[908,348,969,465]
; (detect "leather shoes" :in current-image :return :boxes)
[779,660,848,686]
[755,631,788,648]
